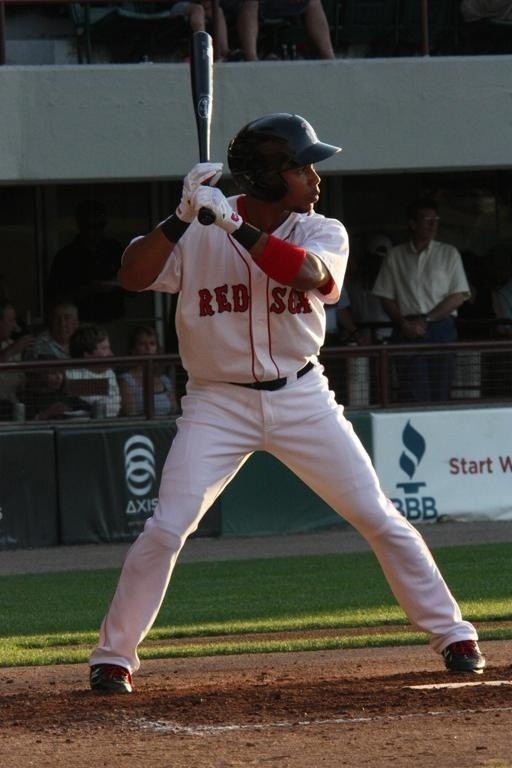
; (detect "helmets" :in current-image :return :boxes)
[227,113,342,200]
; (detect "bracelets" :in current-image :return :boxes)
[158,211,193,245]
[230,221,262,250]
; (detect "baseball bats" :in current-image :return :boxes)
[189,30,216,226]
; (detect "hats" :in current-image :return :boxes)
[367,234,392,256]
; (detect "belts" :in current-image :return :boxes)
[235,362,315,391]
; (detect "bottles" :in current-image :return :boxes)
[281,44,298,59]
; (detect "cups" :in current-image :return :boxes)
[12,403,26,423]
[93,401,106,420]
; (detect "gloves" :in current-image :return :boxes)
[175,161,242,235]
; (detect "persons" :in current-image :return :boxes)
[322,196,511,408]
[165,1,246,65]
[236,0,336,61]
[88,112,489,694]
[1,294,179,425]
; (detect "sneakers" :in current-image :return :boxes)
[90,664,134,694]
[443,642,485,673]
